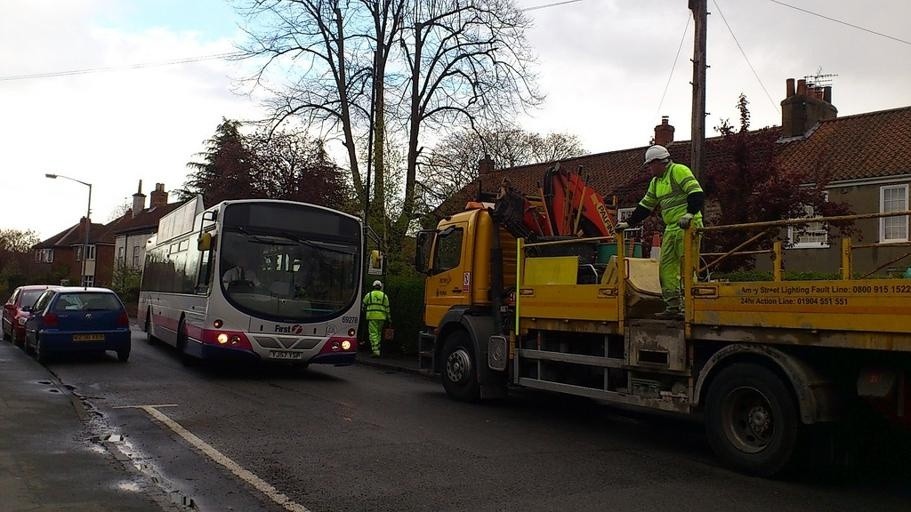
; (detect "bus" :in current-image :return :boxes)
[131,191,385,377]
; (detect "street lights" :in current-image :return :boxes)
[43,172,92,285]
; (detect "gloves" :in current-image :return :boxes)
[677,219,690,229]
[616,221,631,233]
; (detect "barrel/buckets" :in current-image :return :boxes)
[597,242,643,265]
[538,235,598,284]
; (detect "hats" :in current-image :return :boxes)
[373,280,383,287]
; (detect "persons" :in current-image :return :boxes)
[362,280,393,357]
[223,263,261,287]
[613,144,705,319]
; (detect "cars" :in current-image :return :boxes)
[20,285,132,364]
[0,285,89,344]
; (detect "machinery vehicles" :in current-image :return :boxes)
[404,182,911,481]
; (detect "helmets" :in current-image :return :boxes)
[643,145,671,166]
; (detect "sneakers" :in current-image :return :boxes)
[369,350,381,357]
[652,310,686,320]
